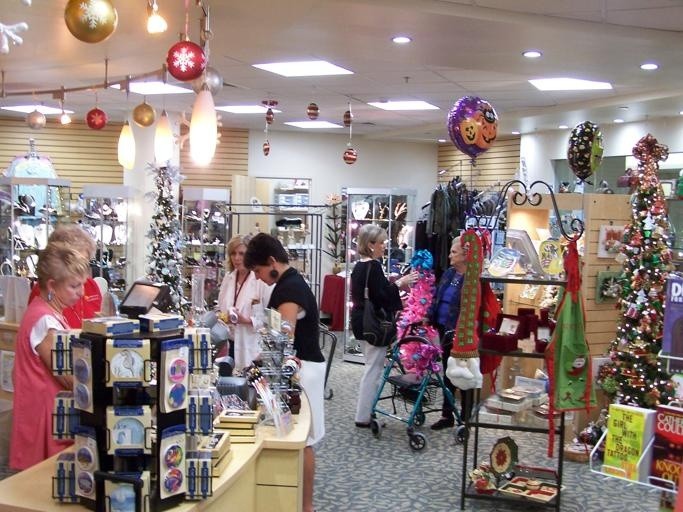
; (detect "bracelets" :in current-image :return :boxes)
[395,280,401,287]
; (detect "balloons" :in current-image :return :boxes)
[444,96,498,195]
[566,120,605,195]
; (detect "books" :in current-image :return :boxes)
[599,395,659,484]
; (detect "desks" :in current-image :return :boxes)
[322,275,345,331]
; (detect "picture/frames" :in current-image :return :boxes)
[199,409,261,477]
[595,271,624,305]
[598,224,625,258]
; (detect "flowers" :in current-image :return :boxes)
[322,192,342,266]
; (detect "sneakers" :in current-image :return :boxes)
[431,415,455,430]
[355,420,386,428]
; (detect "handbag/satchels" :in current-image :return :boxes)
[362,260,398,348]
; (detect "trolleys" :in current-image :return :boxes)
[371,320,469,450]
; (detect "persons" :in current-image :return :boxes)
[5,241,107,472]
[348,223,420,428]
[241,233,328,511]
[214,235,277,372]
[423,235,493,430]
[44,223,108,331]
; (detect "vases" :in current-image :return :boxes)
[333,267,341,274]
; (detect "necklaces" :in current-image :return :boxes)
[67,286,86,326]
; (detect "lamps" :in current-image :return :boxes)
[60,97,72,125]
[189,70,218,167]
[117,75,135,169]
[147,0,168,33]
[154,63,174,166]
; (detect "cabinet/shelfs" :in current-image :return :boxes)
[0,177,135,312]
[342,187,416,367]
[231,212,322,320]
[181,186,230,307]
[461,179,585,510]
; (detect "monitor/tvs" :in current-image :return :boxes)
[118,280,170,319]
